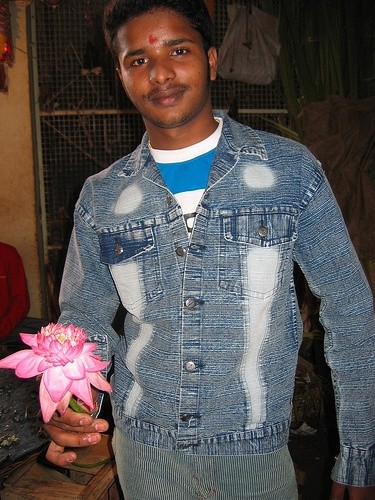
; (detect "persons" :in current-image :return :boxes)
[0,241,31,343]
[44,0,375,500]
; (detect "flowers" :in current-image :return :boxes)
[0,323,112,423]
